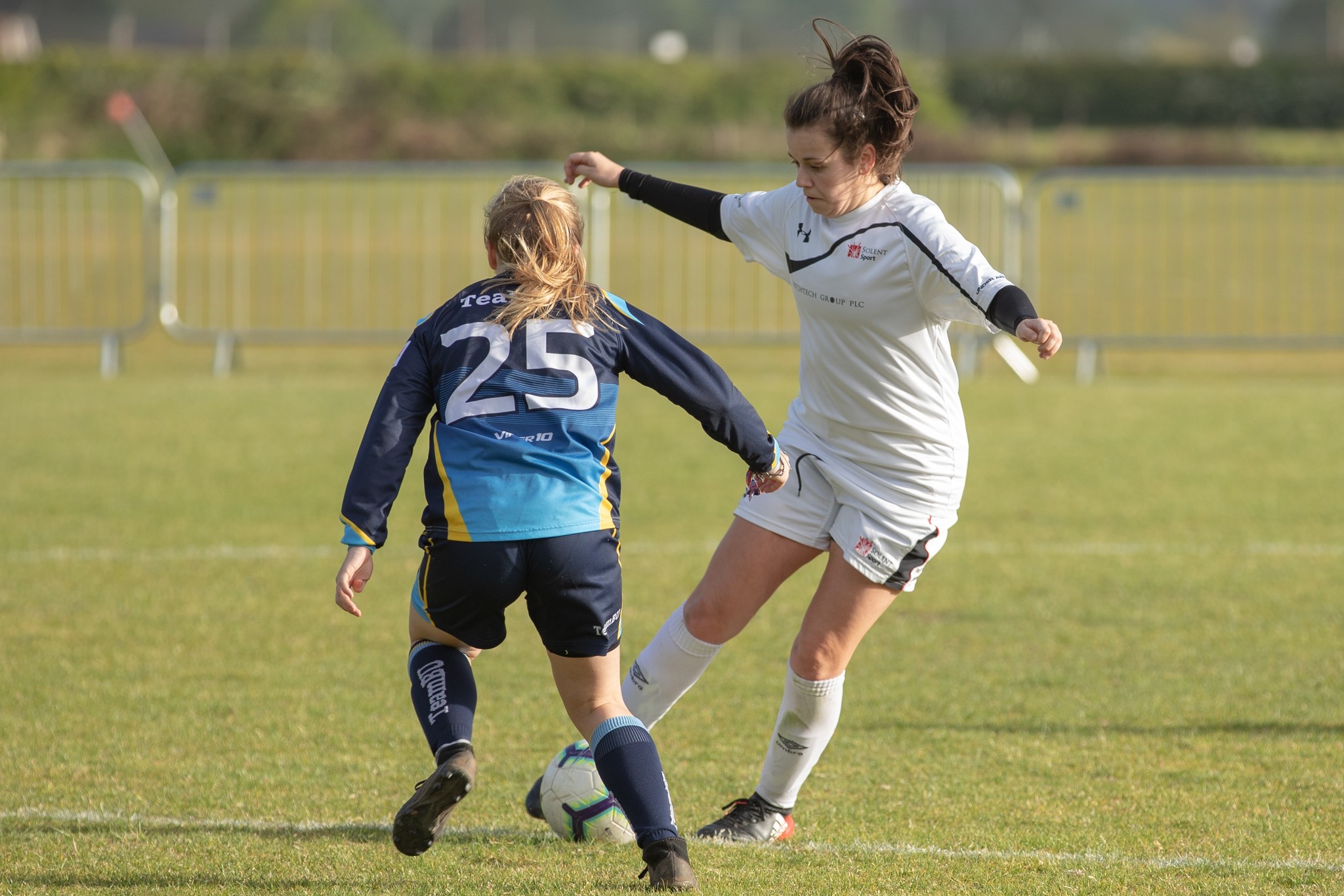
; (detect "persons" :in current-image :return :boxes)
[335,178,792,892]
[524,15,1063,847]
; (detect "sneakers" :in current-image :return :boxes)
[525,773,548,821]
[698,796,797,845]
[391,750,476,858]
[638,837,697,890]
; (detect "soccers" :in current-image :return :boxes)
[539,738,671,844]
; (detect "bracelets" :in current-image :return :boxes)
[742,458,785,504]
